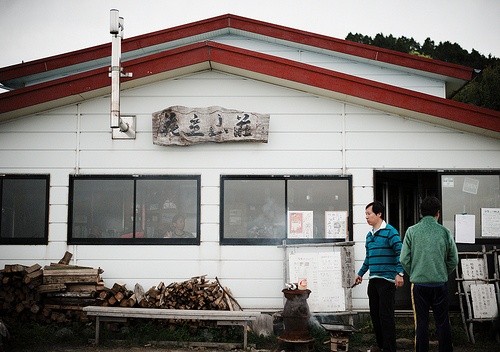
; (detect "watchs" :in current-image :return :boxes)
[398,272,404,277]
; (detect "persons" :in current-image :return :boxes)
[354,202,405,352]
[399,197,459,352]
[163,214,195,238]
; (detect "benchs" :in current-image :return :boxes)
[83,304,261,350]
